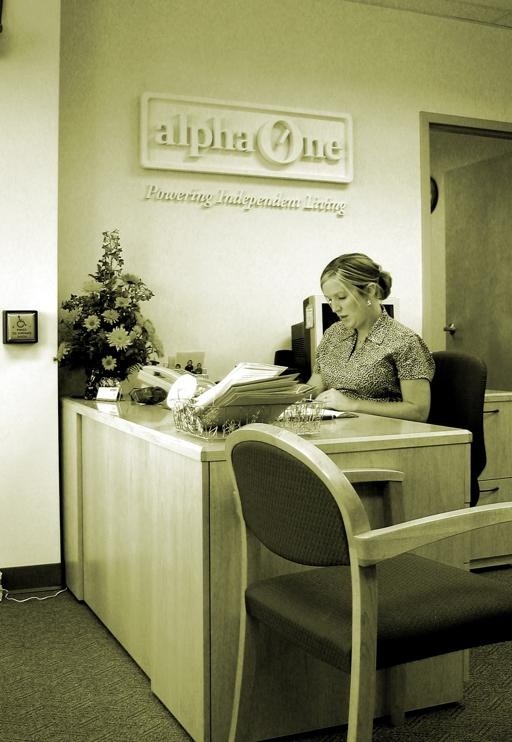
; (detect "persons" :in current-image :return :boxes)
[306,254,436,422]
[175,359,203,373]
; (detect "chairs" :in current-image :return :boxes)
[430,350,488,507]
[224,422,512,741]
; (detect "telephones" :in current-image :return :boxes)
[137,365,204,393]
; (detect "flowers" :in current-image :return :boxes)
[53,228,167,380]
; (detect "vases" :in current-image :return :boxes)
[83,366,123,402]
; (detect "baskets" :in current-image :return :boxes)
[168,395,328,441]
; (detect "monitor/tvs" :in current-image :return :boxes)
[291,294,399,381]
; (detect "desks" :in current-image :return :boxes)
[60,395,473,742]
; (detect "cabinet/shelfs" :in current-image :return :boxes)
[469,390,512,574]
